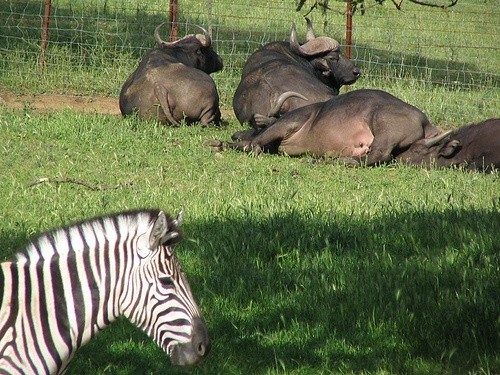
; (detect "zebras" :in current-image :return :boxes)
[0,208,212,375]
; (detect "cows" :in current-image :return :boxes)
[395,118,500,176]
[120,21,223,129]
[233,17,361,126]
[209,89,440,168]
